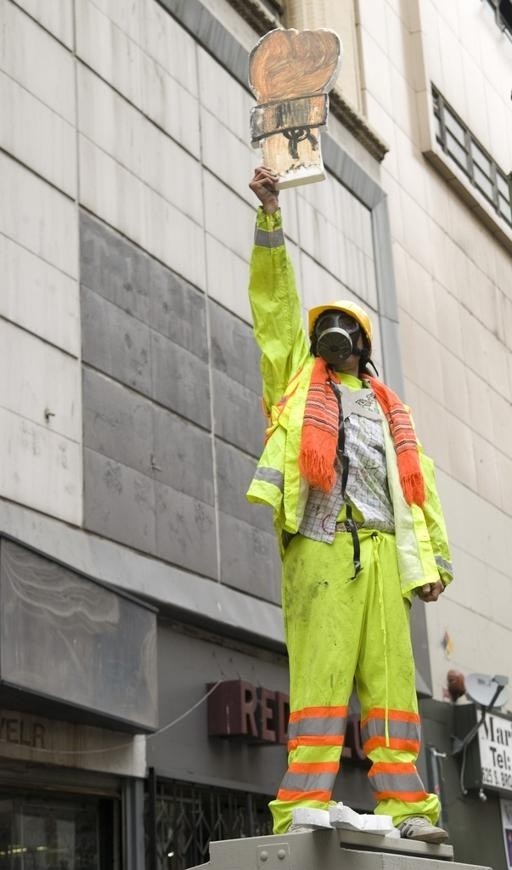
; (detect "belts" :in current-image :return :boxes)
[334,519,364,533]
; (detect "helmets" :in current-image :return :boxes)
[306,298,374,365]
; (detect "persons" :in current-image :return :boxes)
[247,164,452,848]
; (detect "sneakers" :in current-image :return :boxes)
[392,814,452,847]
